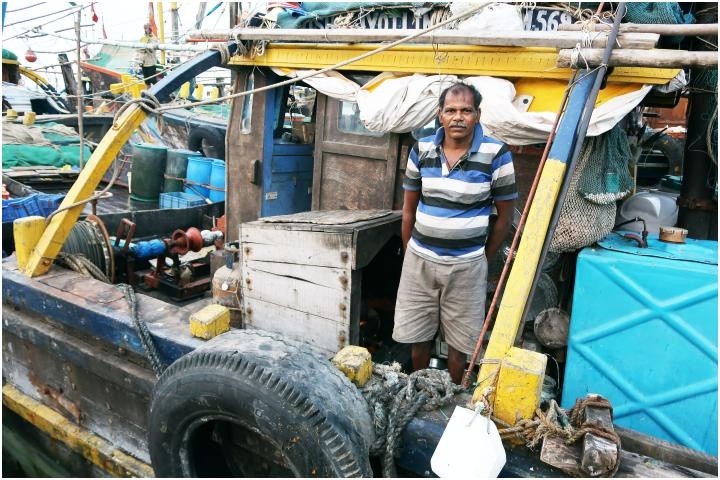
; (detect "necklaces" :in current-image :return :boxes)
[441,143,465,172]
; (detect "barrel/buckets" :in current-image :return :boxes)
[210,162,226,202]
[185,157,223,196]
[164,148,202,193]
[129,145,170,201]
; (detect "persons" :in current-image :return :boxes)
[137,23,159,89]
[391,82,517,388]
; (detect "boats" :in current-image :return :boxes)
[2,1,715,478]
